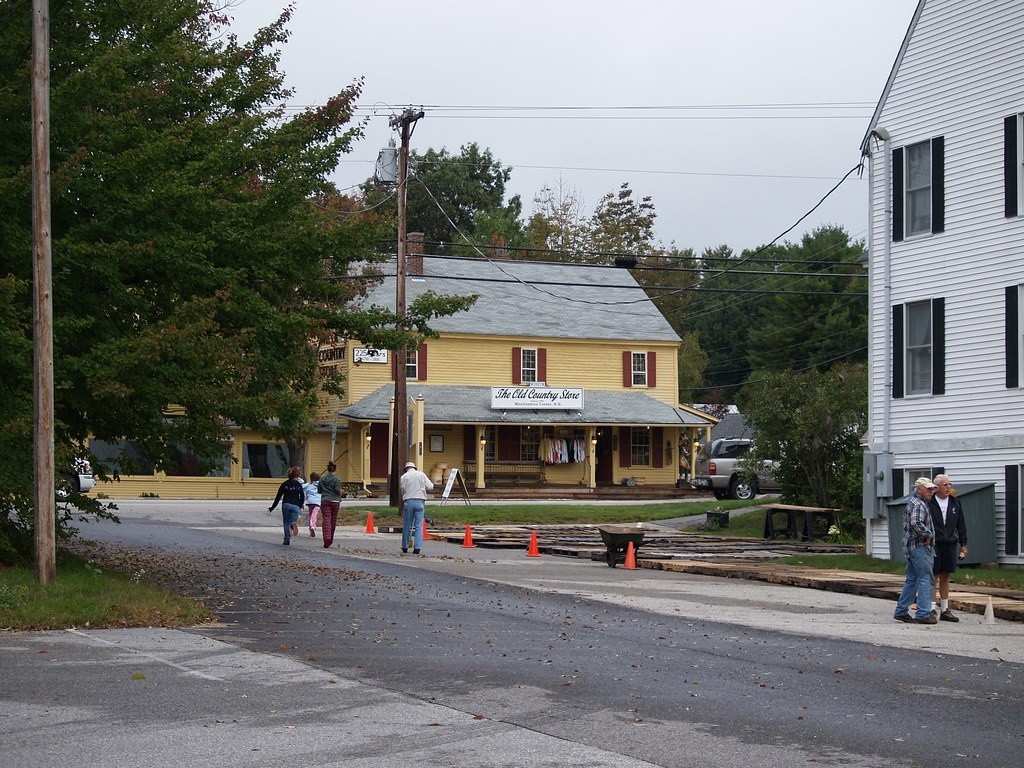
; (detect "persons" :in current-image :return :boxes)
[302,472,322,537]
[267,466,305,545]
[398,462,434,554]
[318,461,342,548]
[893,477,938,624]
[926,473,969,625]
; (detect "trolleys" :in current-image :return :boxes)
[597,523,660,566]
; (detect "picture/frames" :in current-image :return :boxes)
[430,435,444,452]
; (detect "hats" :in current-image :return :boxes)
[915,477,938,489]
[404,462,417,470]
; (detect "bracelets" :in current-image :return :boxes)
[961,544,967,547]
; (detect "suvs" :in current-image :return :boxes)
[695,438,786,501]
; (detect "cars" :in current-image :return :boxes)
[55,457,96,502]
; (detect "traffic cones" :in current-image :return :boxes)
[423,519,431,541]
[621,541,638,569]
[462,524,477,548]
[362,513,377,533]
[979,595,998,626]
[526,532,540,556]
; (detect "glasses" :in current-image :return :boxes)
[943,483,951,486]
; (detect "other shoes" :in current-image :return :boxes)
[324,538,333,548]
[894,614,917,623]
[929,609,937,619]
[283,541,290,545]
[413,549,420,554]
[403,548,407,553]
[940,608,959,622]
[915,616,938,624]
[309,526,316,537]
[292,523,298,537]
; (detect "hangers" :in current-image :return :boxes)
[543,434,585,440]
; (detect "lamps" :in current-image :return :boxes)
[480,435,487,445]
[366,432,372,441]
[591,433,598,444]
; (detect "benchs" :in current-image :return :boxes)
[761,503,842,542]
